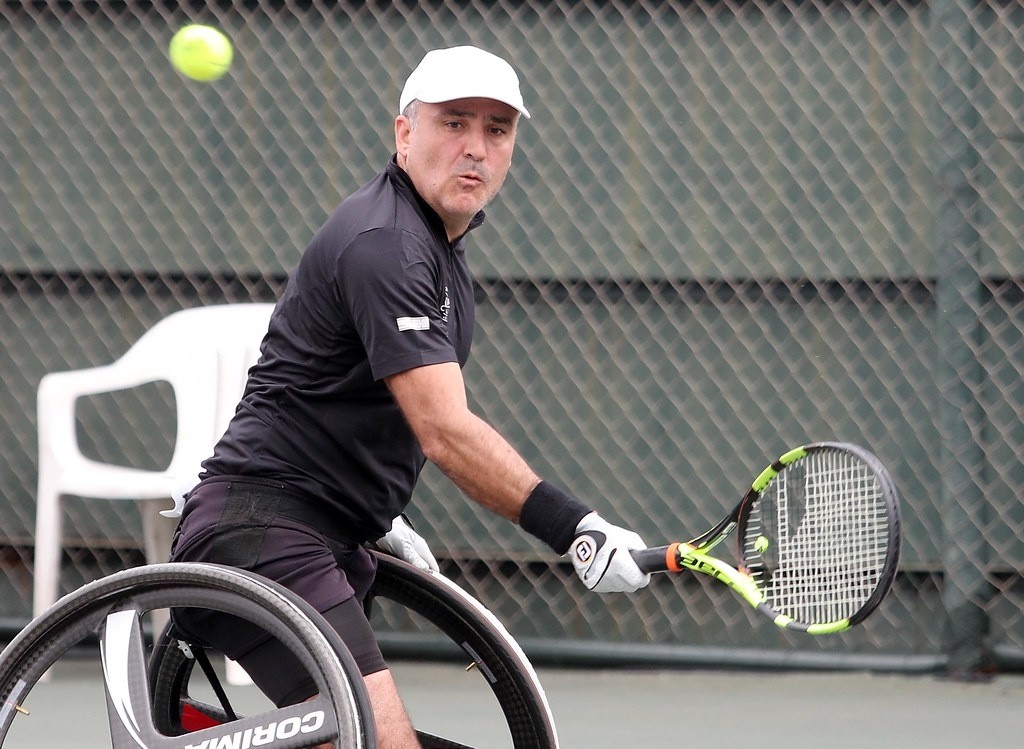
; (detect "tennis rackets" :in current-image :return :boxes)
[621,437,904,637]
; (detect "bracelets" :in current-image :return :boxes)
[520,480,591,556]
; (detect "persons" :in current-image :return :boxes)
[169,44,651,749]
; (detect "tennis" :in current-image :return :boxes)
[166,22,236,85]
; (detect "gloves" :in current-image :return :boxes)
[559,510,651,593]
[375,514,440,573]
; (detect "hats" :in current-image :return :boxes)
[400,46,531,120]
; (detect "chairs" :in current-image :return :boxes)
[30,302,275,686]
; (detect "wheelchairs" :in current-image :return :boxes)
[0,552,559,749]
